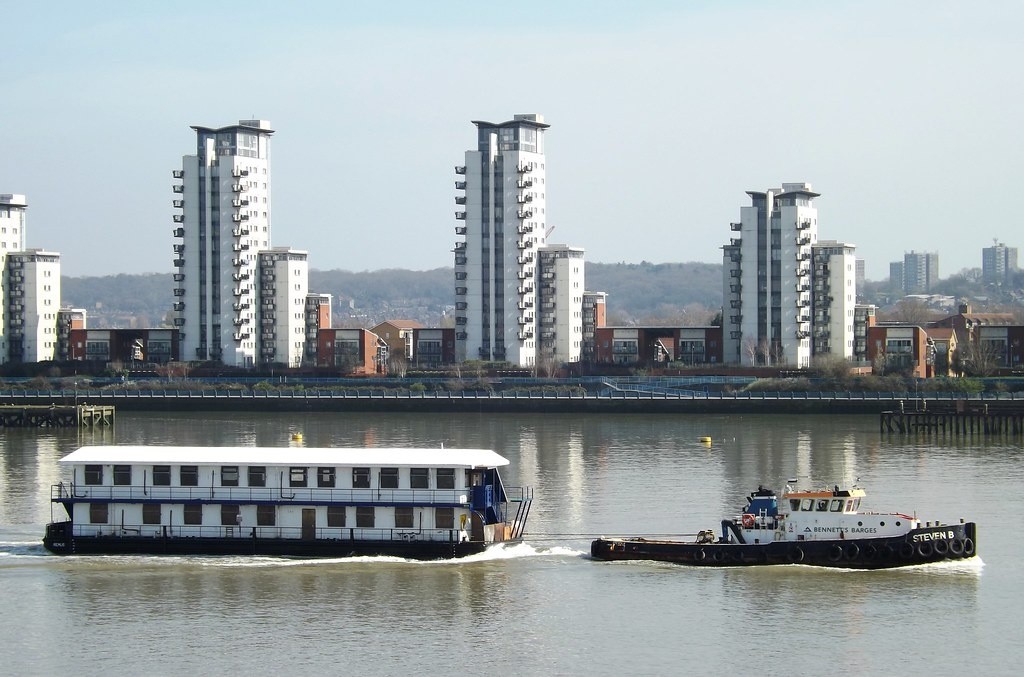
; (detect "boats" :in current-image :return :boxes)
[45,447,533,556]
[590,479,978,568]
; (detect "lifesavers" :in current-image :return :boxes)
[843,543,860,561]
[934,539,948,556]
[901,543,914,558]
[949,538,963,556]
[733,550,743,561]
[694,550,705,560]
[713,551,725,561]
[827,545,843,561]
[863,545,878,563]
[963,537,974,554]
[742,514,755,526]
[878,546,893,562]
[918,541,934,558]
[788,546,804,563]
